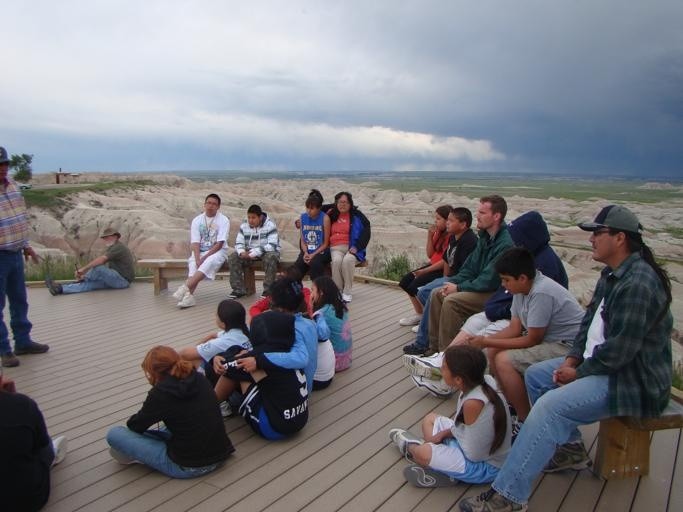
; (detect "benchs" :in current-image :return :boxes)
[135,252,333,297]
[593,394,683,484]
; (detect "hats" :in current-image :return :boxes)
[579,205,643,235]
[0,146,12,164]
[100,228,121,240]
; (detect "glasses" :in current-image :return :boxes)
[592,228,608,236]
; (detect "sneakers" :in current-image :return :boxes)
[389,427,424,455]
[109,447,142,466]
[14,341,49,355]
[172,284,197,309]
[542,440,592,472]
[510,418,523,447]
[45,275,63,296]
[399,313,422,333]
[403,465,460,488]
[260,289,269,297]
[49,435,69,468]
[2,353,19,368]
[403,343,452,399]
[219,399,231,415]
[228,291,241,297]
[459,489,524,512]
[342,292,353,302]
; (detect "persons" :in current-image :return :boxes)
[389,196,518,357]
[227,204,281,302]
[0,372,65,512]
[464,247,588,445]
[388,344,514,491]
[179,276,354,444]
[295,192,370,303]
[459,205,674,511]
[283,188,332,284]
[45,228,136,295]
[172,193,232,309]
[106,343,237,480]
[1,146,49,367]
[403,213,570,401]
